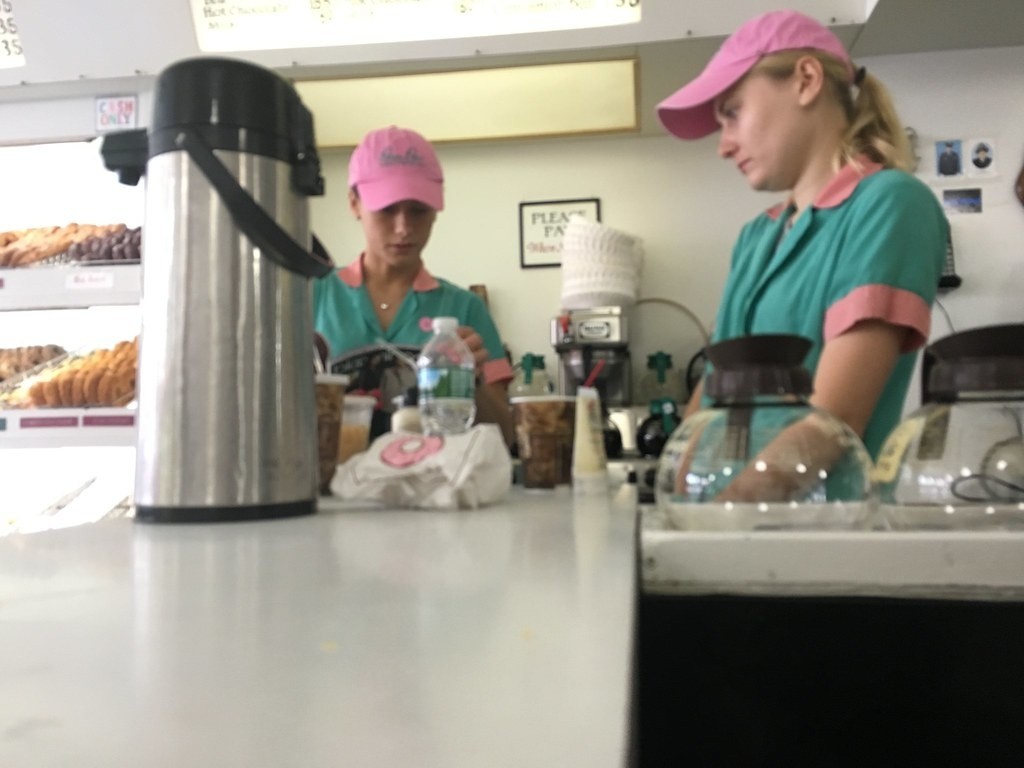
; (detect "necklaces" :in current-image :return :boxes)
[380,303,389,309]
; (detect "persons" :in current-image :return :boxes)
[652,10,948,503]
[311,126,515,448]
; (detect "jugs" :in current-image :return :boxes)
[874,322,1024,533]
[642,351,685,405]
[507,353,554,397]
[654,330,882,532]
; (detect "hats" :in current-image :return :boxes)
[656,9,845,140]
[347,128,443,214]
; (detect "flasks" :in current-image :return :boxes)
[132,55,335,523]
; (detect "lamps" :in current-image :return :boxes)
[295,58,642,147]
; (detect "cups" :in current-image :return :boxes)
[509,395,577,495]
[569,386,608,494]
[338,394,378,466]
[313,373,350,491]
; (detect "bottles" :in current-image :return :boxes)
[415,316,477,438]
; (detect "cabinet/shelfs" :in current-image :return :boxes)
[1,258,143,452]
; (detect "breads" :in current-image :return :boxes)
[0,222,140,407]
